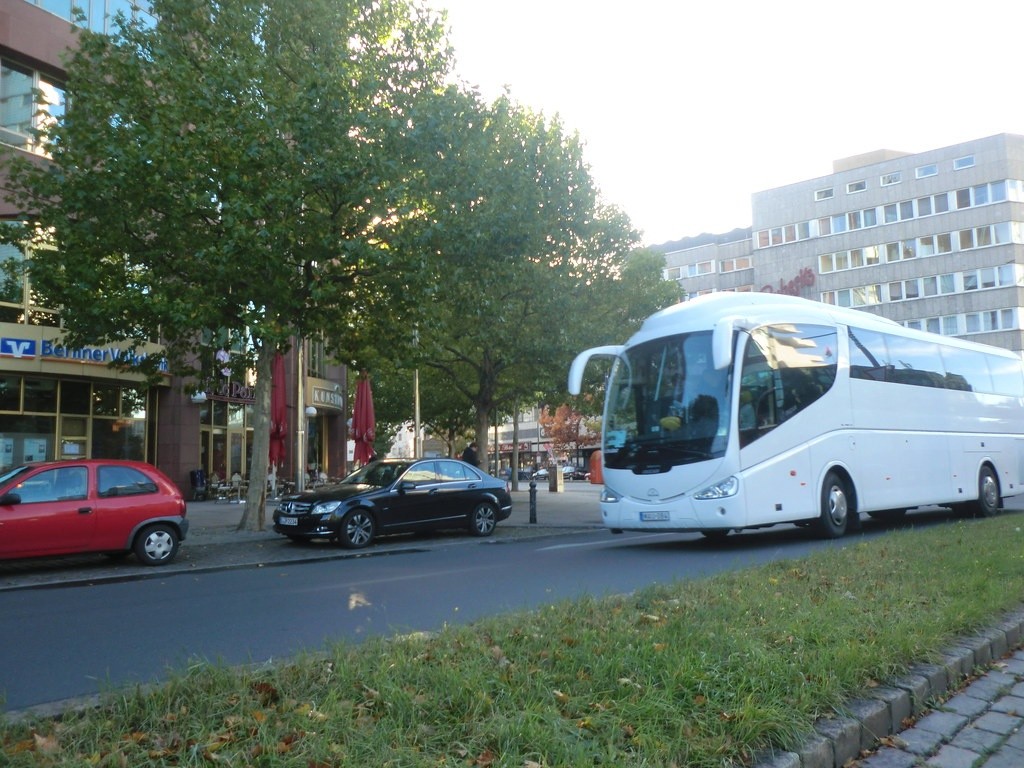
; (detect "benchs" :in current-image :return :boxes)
[228,480,249,505]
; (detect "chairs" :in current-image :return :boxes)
[67,472,85,499]
[210,472,227,498]
[230,473,247,500]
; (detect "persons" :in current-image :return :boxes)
[316,466,328,481]
[739,388,757,428]
[231,472,242,487]
[461,443,480,468]
[210,472,221,487]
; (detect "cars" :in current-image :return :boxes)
[505,466,591,481]
[273,458,512,549]
[0,459,190,566]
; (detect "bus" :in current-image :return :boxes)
[568,292,1024,540]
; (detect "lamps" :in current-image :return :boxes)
[304,404,321,418]
[190,388,208,404]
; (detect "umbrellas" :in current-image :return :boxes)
[350,367,377,468]
[268,349,288,493]
